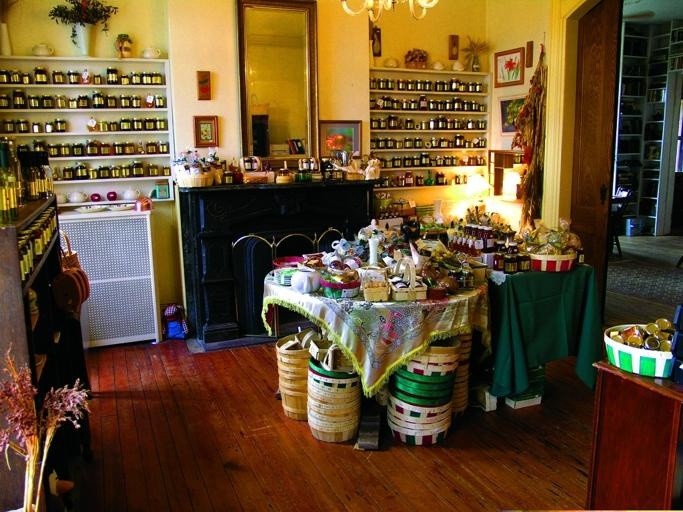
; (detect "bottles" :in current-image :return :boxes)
[379,118,387,130]
[477,157,484,166]
[388,79,395,90]
[100,142,111,155]
[78,94,89,109]
[94,74,102,85]
[378,137,386,149]
[393,98,401,110]
[252,158,258,171]
[466,139,474,148]
[152,72,163,84]
[478,137,485,148]
[376,96,384,110]
[384,95,392,110]
[422,153,430,167]
[437,99,445,110]
[98,165,110,179]
[444,156,452,166]
[468,81,476,92]
[86,116,98,132]
[380,78,387,90]
[477,119,486,130]
[413,156,421,167]
[467,118,475,130]
[156,117,166,131]
[476,82,483,93]
[437,171,447,186]
[107,94,117,108]
[425,80,432,91]
[223,170,235,185]
[405,171,414,187]
[414,137,423,148]
[444,81,450,92]
[494,253,504,271]
[121,93,131,108]
[415,174,424,186]
[403,138,413,148]
[63,165,73,180]
[82,68,91,85]
[89,168,98,179]
[463,174,468,184]
[113,141,123,155]
[504,253,517,274]
[68,69,79,85]
[435,80,444,91]
[496,241,507,254]
[27,94,41,109]
[421,120,429,130]
[371,118,379,130]
[388,114,398,129]
[464,223,473,236]
[460,81,467,92]
[163,164,170,177]
[74,163,89,181]
[455,134,465,147]
[410,99,418,111]
[72,143,84,157]
[85,139,98,157]
[404,156,412,168]
[390,175,397,187]
[92,90,106,109]
[394,156,402,168]
[144,117,154,130]
[370,77,377,89]
[4,118,15,133]
[13,89,26,109]
[575,246,585,268]
[517,252,530,272]
[464,100,471,111]
[110,165,122,178]
[18,118,29,133]
[69,97,78,109]
[430,100,437,110]
[447,119,454,130]
[382,176,389,188]
[429,118,437,130]
[454,97,462,111]
[120,118,131,131]
[59,142,70,157]
[53,70,64,84]
[407,79,415,91]
[437,115,447,130]
[453,156,460,166]
[0,94,11,110]
[125,141,136,154]
[45,121,54,133]
[449,140,455,148]
[22,72,30,85]
[147,140,158,153]
[417,79,424,90]
[396,139,403,149]
[134,117,142,131]
[477,225,484,240]
[454,118,461,129]
[55,119,66,133]
[445,99,454,110]
[483,226,494,253]
[479,104,485,112]
[34,66,48,85]
[472,224,478,237]
[109,121,118,131]
[26,138,58,202]
[107,66,118,85]
[333,168,344,181]
[452,78,460,92]
[54,94,67,109]
[0,136,26,224]
[155,94,165,108]
[385,158,393,168]
[16,206,58,288]
[142,72,152,85]
[440,137,449,148]
[148,164,159,177]
[455,173,461,185]
[0,69,10,83]
[145,91,154,108]
[131,72,141,85]
[473,100,479,111]
[425,169,434,186]
[12,69,20,84]
[370,97,376,110]
[507,242,518,255]
[121,165,130,177]
[468,155,476,166]
[244,157,252,171]
[402,99,410,110]
[405,118,414,130]
[397,79,406,90]
[99,120,109,132]
[121,74,130,85]
[130,161,145,178]
[436,156,444,167]
[431,138,439,148]
[397,176,405,187]
[386,137,394,149]
[160,141,168,153]
[41,95,53,109]
[31,121,41,134]
[132,94,141,109]
[418,95,428,111]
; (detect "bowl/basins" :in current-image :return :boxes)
[603,323,675,378]
[529,249,577,272]
[320,277,361,298]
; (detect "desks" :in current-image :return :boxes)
[485,262,603,410]
[261,267,492,451]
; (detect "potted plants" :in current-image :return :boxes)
[48,0,119,58]
[460,34,494,72]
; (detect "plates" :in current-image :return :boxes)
[74,205,108,214]
[273,270,291,286]
[108,204,136,211]
[272,256,304,267]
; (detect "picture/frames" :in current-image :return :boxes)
[193,115,219,148]
[494,47,525,88]
[318,120,362,159]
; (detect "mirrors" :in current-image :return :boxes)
[234,0,320,168]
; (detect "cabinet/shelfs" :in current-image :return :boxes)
[637,19,673,236]
[586,357,683,511]
[0,55,188,322]
[370,67,493,234]
[58,207,159,345]
[497,94,528,136]
[612,20,653,234]
[668,18,683,72]
[0,191,93,512]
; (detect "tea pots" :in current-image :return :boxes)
[123,187,140,200]
[143,47,161,58]
[31,44,54,56]
[291,268,320,294]
[67,191,88,203]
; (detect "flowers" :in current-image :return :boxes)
[0,349,93,511]
